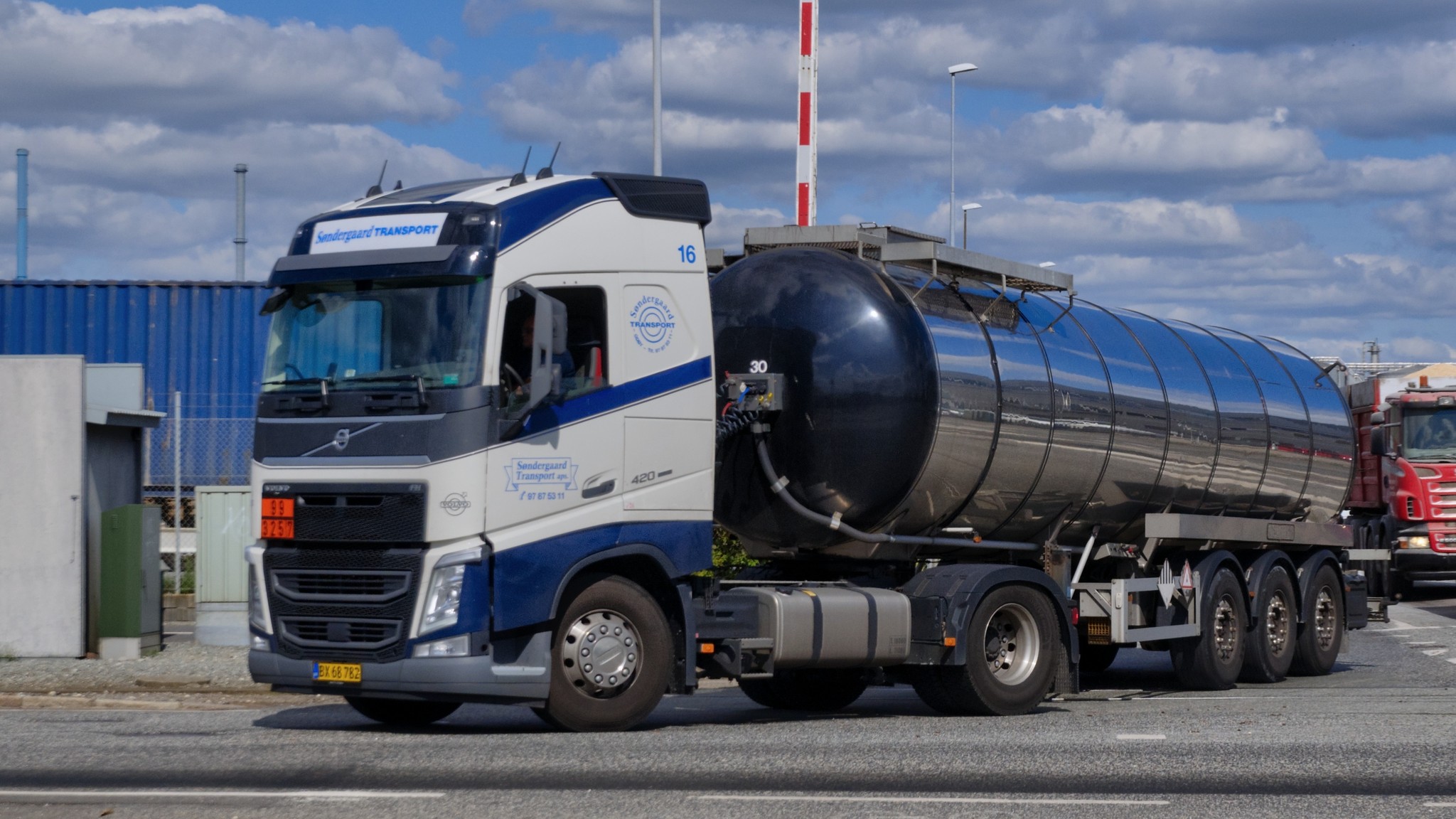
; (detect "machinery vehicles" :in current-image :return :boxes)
[241,139,1396,738]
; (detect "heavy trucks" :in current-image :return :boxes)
[1330,338,1456,602]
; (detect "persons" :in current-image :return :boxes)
[498,306,575,406]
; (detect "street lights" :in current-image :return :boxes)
[963,203,983,249]
[949,63,978,245]
